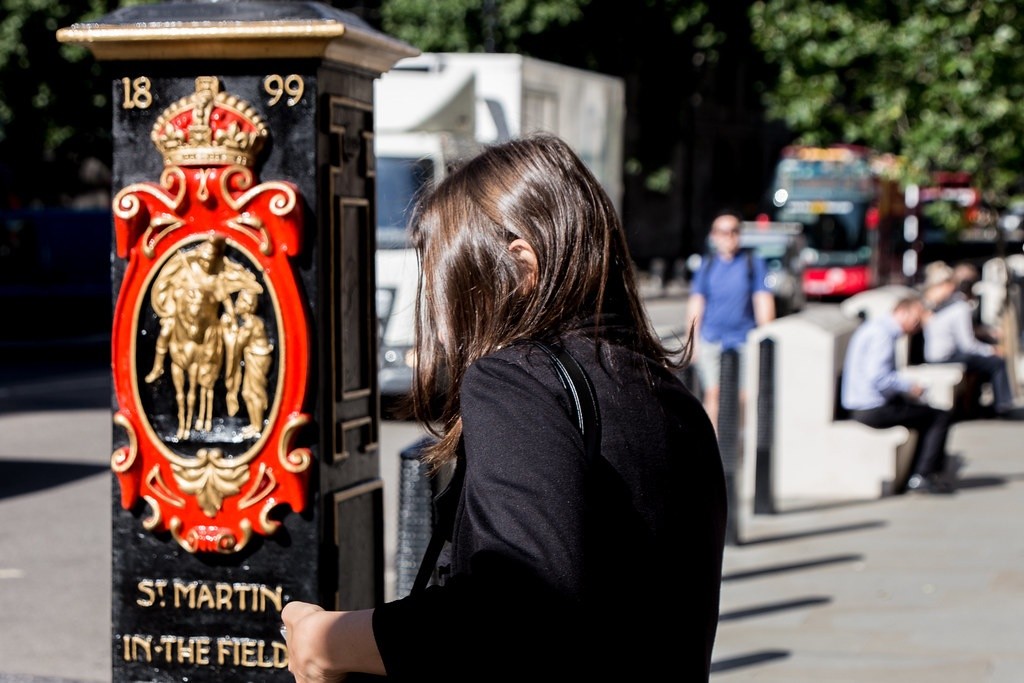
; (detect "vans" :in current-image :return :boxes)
[708,222,804,314]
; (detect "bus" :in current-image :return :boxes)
[754,142,923,295]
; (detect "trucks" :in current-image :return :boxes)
[374,53,627,416]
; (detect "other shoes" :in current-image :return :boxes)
[894,476,953,493]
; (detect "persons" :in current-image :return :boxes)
[840,265,1023,491]
[282,132,728,683]
[686,215,778,436]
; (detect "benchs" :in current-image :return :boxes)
[750,285,918,497]
